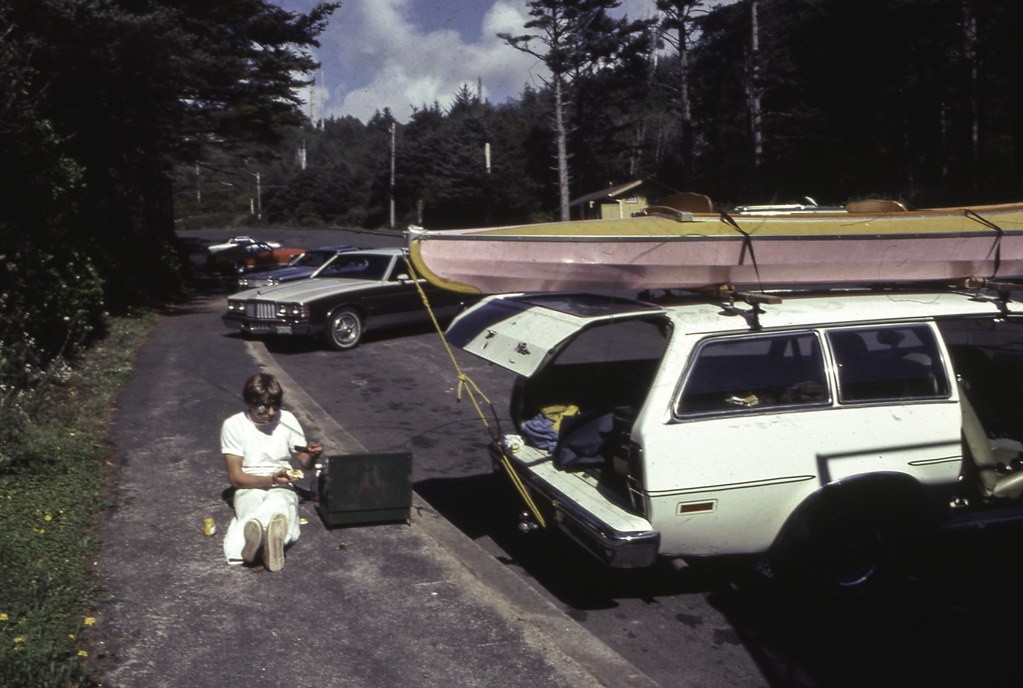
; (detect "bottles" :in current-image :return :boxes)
[203,518,217,536]
[310,462,322,501]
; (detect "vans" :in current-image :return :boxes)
[440,282,1022,616]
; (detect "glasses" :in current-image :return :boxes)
[251,400,280,411]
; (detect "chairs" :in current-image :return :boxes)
[900,351,1023,499]
[807,332,875,369]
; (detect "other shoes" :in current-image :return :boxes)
[242,518,265,565]
[264,514,287,572]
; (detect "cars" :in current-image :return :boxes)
[187,235,490,349]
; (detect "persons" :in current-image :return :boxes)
[221,373,324,573]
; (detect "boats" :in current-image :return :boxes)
[404,190,1022,299]
